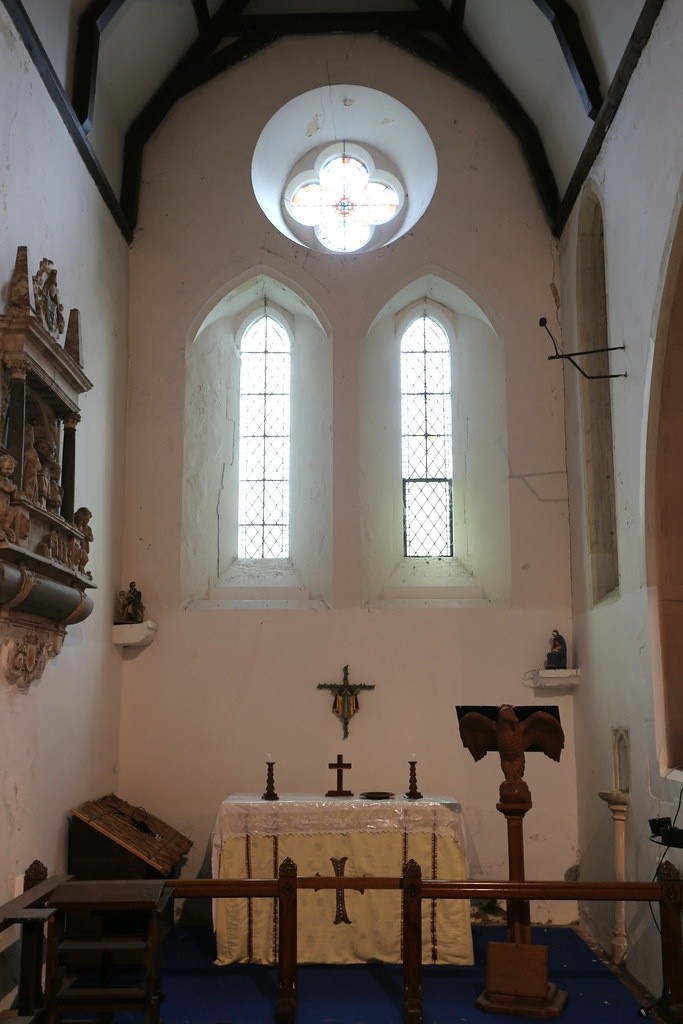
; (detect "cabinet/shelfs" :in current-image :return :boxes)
[46,879,176,1024]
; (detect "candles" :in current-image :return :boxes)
[412,753,416,761]
[267,753,271,762]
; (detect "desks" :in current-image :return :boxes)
[211,793,483,966]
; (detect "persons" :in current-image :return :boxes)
[0,455,18,529]
[551,630,567,668]
[73,507,93,573]
[126,581,140,620]
[24,423,61,504]
[339,681,351,717]
